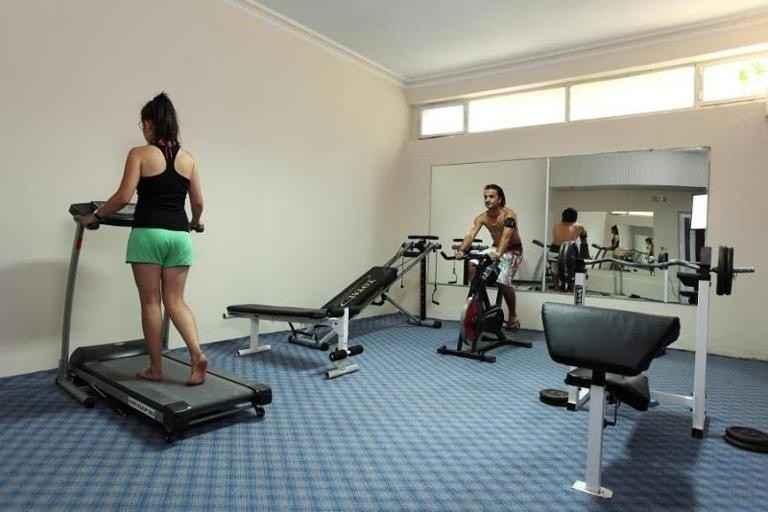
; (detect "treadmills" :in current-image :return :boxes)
[56,201,272,442]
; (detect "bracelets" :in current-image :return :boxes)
[92,208,103,219]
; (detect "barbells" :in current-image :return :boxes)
[548,240,754,297]
[657,246,711,273]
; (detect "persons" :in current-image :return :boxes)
[544,206,586,291]
[452,183,523,334]
[643,238,653,275]
[603,223,619,270]
[79,89,208,387]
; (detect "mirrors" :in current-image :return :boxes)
[428,148,708,308]
